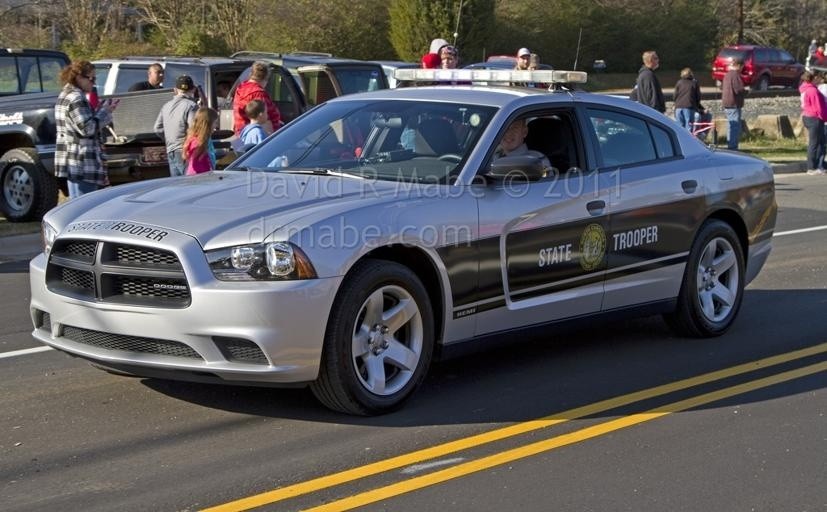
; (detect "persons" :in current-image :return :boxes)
[397,37,547,88]
[152,72,201,178]
[693,103,711,143]
[55,60,120,197]
[797,39,826,174]
[230,62,282,151]
[670,68,701,134]
[635,51,667,113]
[215,78,233,110]
[180,108,219,177]
[629,79,640,102]
[480,116,556,181]
[719,58,750,152]
[231,97,269,160]
[127,63,164,96]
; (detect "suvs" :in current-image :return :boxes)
[1,48,561,224]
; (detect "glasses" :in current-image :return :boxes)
[82,76,97,83]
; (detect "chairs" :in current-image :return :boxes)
[522,118,578,175]
[415,119,457,164]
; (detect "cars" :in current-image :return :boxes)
[27,84,779,414]
[714,46,812,93]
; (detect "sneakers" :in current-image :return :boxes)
[806,168,827,175]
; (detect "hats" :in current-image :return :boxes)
[517,48,531,57]
[442,45,457,55]
[422,53,441,68]
[176,76,195,92]
